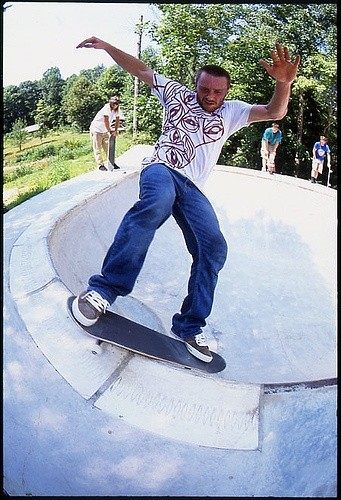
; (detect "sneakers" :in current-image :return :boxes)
[311,177,314,182]
[99,165,107,170]
[113,163,120,169]
[169,327,212,363]
[314,178,316,183]
[72,288,109,327]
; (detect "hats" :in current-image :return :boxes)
[109,97,119,103]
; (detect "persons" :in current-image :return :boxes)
[89,95,120,171]
[260,123,283,172]
[72,36,300,363]
[311,135,331,183]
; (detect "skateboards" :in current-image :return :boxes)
[66,295,226,374]
[107,135,116,172]
[327,167,334,187]
